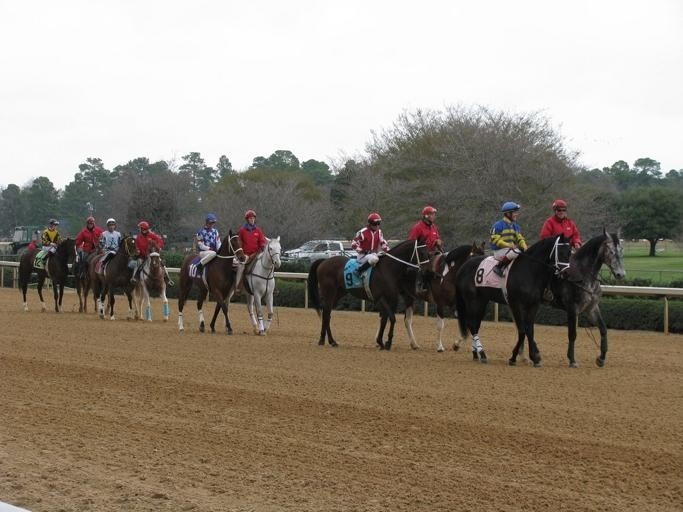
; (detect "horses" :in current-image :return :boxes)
[306,232,434,351]
[177,229,246,335]
[127,241,170,322]
[453,232,576,368]
[375,239,487,352]
[512,222,627,368]
[18,237,79,314]
[71,260,111,318]
[223,235,282,336]
[89,231,141,321]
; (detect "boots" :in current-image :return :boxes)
[354,261,371,280]
[42,251,53,264]
[492,255,512,277]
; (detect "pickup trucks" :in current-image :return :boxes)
[281,238,359,265]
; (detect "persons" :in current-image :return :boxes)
[407,206,445,256]
[351,212,391,279]
[188,212,224,280]
[41,218,63,261]
[235,209,280,296]
[74,216,105,265]
[130,221,175,290]
[489,201,529,276]
[95,218,124,315]
[540,198,583,257]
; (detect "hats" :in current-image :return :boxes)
[421,205,438,216]
[552,199,568,210]
[137,221,150,230]
[86,216,96,226]
[205,213,217,224]
[106,218,117,227]
[244,209,257,220]
[368,213,382,225]
[502,202,521,214]
[49,218,60,225]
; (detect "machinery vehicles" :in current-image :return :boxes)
[0,224,47,263]
